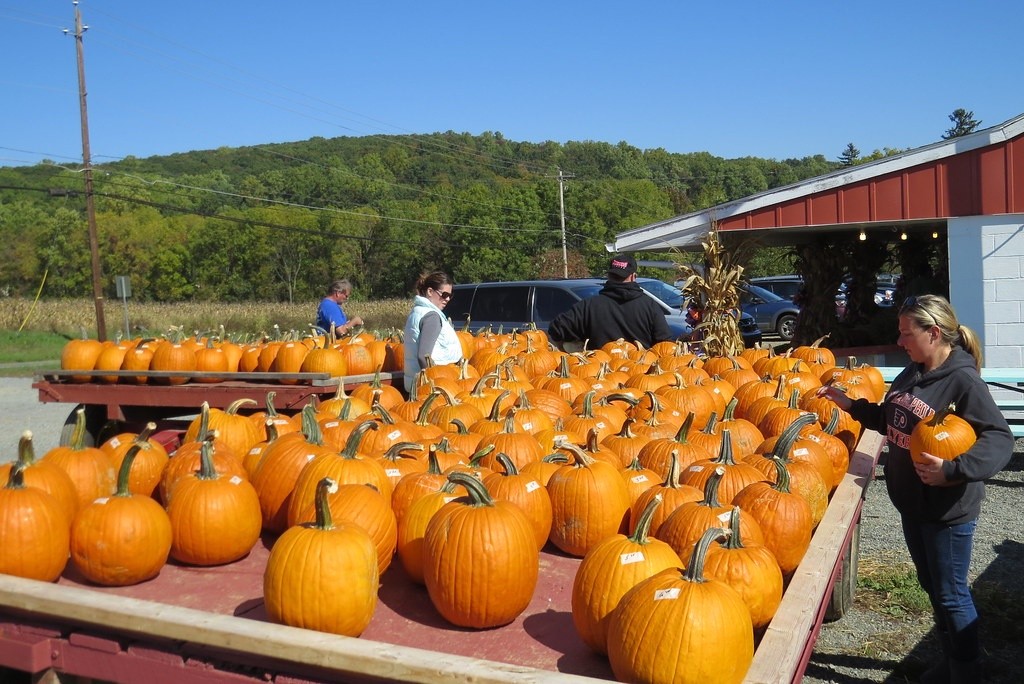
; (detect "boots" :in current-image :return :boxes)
[949,656,984,684]
[920,631,955,684]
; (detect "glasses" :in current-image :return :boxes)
[903,296,938,325]
[435,288,453,299]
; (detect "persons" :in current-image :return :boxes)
[816,294,1015,684]
[317,279,362,339]
[404,272,464,402]
[549,256,674,351]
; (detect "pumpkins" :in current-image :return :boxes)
[910,402,977,465]
[1,334,885,683]
[61,316,547,385]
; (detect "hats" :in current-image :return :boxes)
[603,255,637,278]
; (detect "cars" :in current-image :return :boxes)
[739,284,801,342]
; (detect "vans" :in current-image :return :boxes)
[750,273,901,326]
[440,278,763,351]
